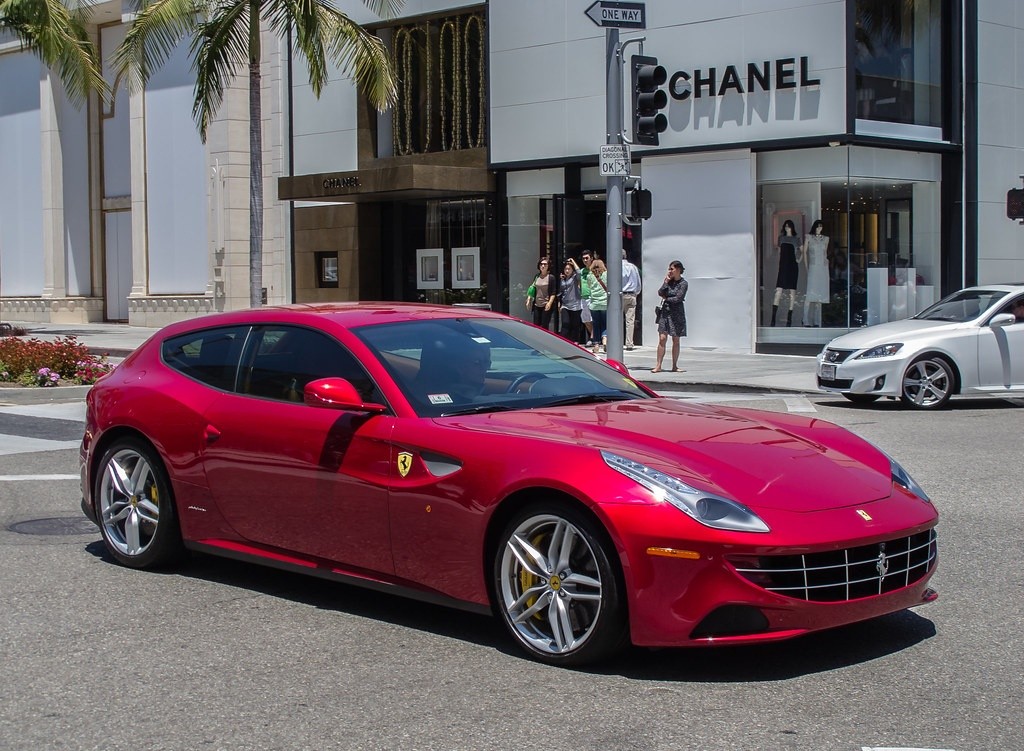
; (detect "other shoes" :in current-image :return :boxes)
[593,344,599,353]
[603,344,607,352]
[627,347,633,351]
[585,338,594,348]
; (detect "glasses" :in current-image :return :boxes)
[582,257,588,260]
[464,358,492,367]
[669,268,673,270]
[1011,305,1020,310]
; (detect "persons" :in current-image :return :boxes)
[440,345,498,403]
[835,252,864,323]
[771,219,830,327]
[526,249,642,353]
[651,261,689,373]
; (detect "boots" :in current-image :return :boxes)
[786,310,793,327]
[770,305,778,327]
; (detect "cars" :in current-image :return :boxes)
[816,282,1024,412]
[77,300,940,669]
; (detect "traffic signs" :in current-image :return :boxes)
[584,0,645,29]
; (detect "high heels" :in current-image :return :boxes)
[801,320,819,327]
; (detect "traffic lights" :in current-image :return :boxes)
[631,54,669,147]
[1006,187,1024,222]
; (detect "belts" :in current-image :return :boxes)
[623,292,634,294]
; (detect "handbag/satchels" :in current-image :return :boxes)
[527,276,538,297]
[655,306,660,324]
